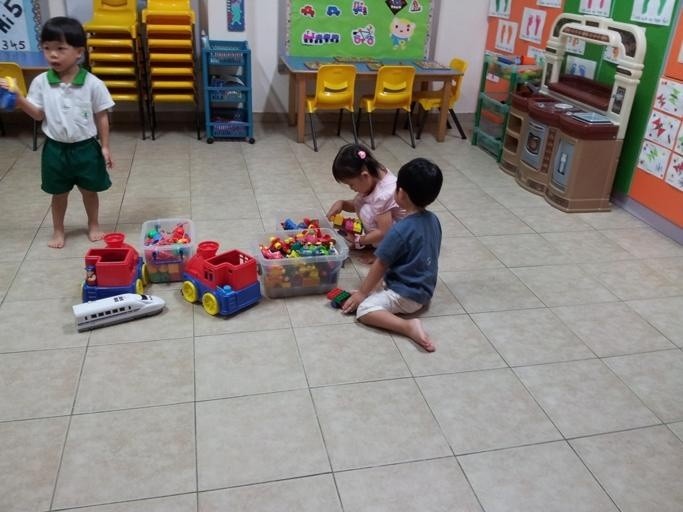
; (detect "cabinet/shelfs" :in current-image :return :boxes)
[471,49,543,159]
[198,32,257,147]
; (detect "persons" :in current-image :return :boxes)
[341,157,444,354]
[323,142,407,264]
[0,15,117,248]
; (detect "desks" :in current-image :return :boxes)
[275,54,466,146]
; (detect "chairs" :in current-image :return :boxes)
[0,59,46,152]
[409,56,470,141]
[303,59,363,152]
[76,1,202,142]
[354,63,422,150]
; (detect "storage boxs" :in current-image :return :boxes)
[250,226,352,304]
[137,214,196,287]
[273,205,336,235]
